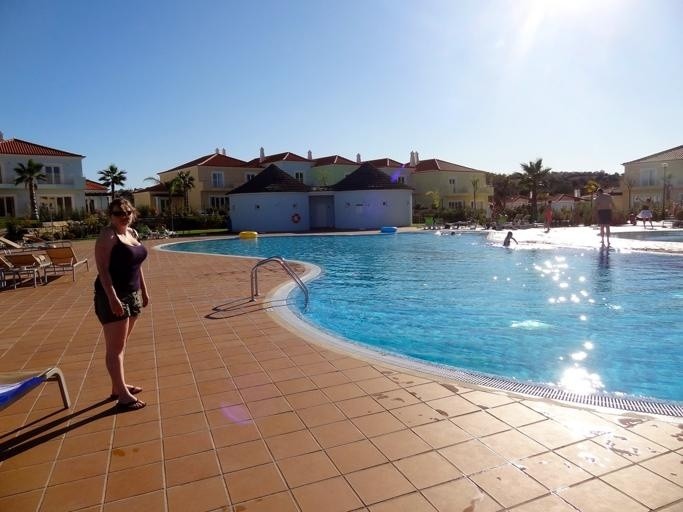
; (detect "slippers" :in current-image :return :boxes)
[114,397,147,409]
[109,385,143,398]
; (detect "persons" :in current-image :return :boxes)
[91,197,148,409]
[635,196,653,227]
[503,231,518,247]
[591,188,617,245]
[542,200,553,232]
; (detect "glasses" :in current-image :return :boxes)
[108,209,133,217]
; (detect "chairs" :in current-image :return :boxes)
[0,367,70,411]
[0,239,88,290]
[420,217,475,229]
[504,213,530,225]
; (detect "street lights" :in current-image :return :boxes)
[661,163,668,220]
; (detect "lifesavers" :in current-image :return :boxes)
[380,227,397,233]
[239,231,258,239]
